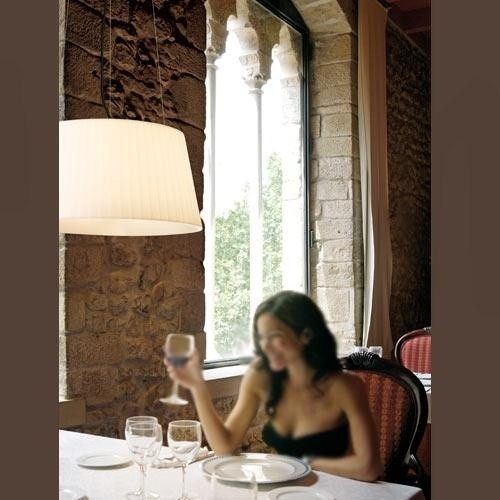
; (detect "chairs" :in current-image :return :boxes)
[334,326,431,499]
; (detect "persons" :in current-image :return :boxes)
[163,290,382,482]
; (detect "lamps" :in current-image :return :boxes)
[57,0,202,236]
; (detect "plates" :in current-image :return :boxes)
[268,485,334,500]
[202,452,314,484]
[74,448,131,469]
[59,481,86,500]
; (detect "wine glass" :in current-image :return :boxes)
[168,420,203,498]
[160,333,195,406]
[353,346,384,359]
[122,416,163,500]
